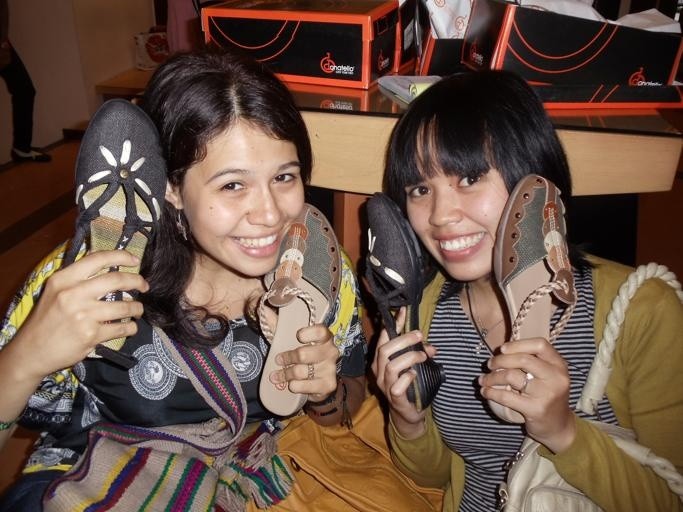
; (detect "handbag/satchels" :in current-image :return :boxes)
[43,420,235,511]
[497,419,682,512]
[233,393,443,511]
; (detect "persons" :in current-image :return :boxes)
[0,47,369,512]
[369,68,682,511]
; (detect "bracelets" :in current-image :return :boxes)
[0,406,29,431]
[307,374,354,432]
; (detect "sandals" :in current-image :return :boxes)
[62,99,167,360]
[360,190,440,412]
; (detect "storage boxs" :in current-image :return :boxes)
[198,1,401,91]
[459,1,682,88]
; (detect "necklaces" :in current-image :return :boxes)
[469,280,508,357]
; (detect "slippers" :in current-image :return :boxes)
[256,205,343,417]
[487,174,578,427]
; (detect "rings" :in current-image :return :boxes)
[519,372,534,393]
[308,362,315,381]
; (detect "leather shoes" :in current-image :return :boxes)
[9,149,52,163]
